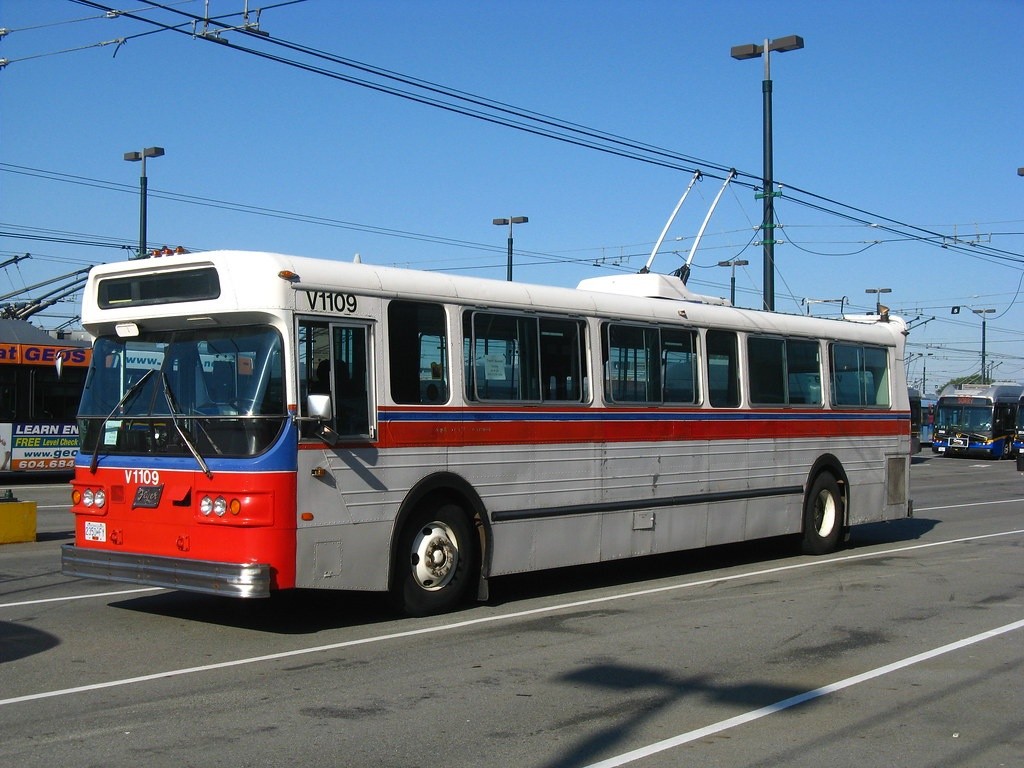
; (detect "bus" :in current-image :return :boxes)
[0,263,232,482]
[907,381,1024,471]
[59,174,914,617]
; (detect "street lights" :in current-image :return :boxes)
[718,260,749,307]
[493,216,528,281]
[973,309,996,385]
[729,34,804,311]
[865,288,892,314]
[123,146,165,256]
